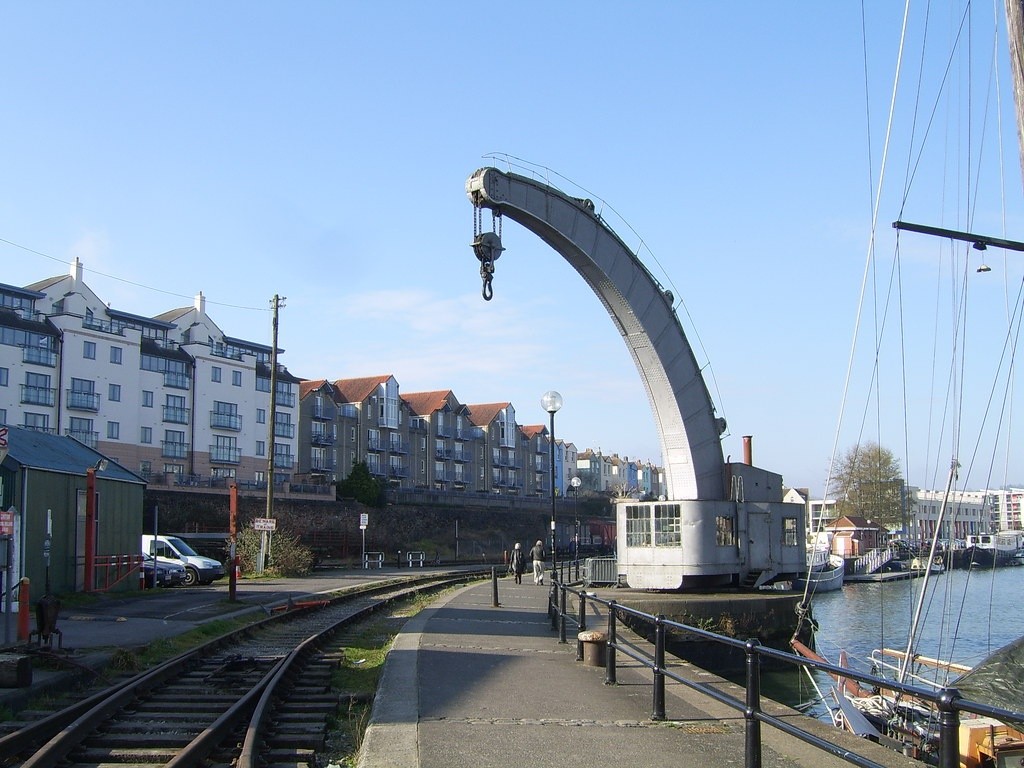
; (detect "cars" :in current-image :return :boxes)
[140,553,187,588]
[886,538,967,551]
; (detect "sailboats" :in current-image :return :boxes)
[790,1,1024,768]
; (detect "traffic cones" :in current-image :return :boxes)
[235,554,244,580]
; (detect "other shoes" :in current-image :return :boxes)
[540,580,543,586]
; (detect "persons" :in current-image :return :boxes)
[529,540,546,585]
[510,543,526,585]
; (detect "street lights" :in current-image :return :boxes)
[539,389,564,575]
[570,475,581,578]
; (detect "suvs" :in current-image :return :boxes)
[140,534,226,586]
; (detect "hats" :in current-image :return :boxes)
[515,543,521,549]
[536,541,543,546]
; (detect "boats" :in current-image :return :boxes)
[908,557,946,572]
[795,552,846,593]
[961,530,1023,568]
[1014,552,1024,566]
